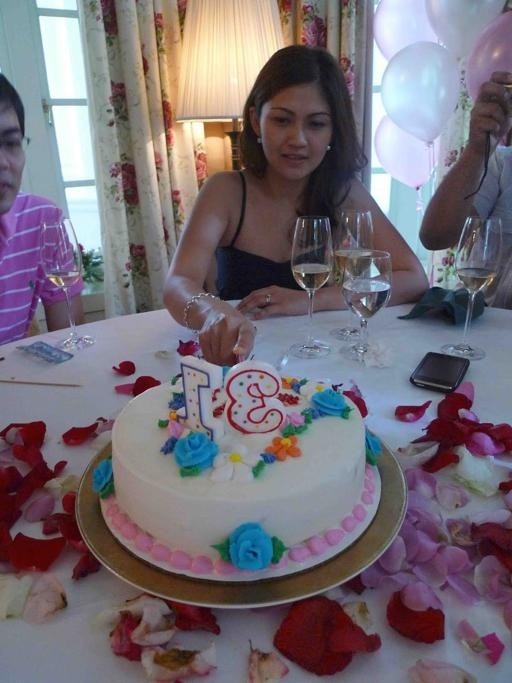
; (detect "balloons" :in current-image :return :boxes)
[374,0,512,191]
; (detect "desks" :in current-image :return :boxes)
[4,296,511,683]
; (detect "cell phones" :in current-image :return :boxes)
[409,352,470,393]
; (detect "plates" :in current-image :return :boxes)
[74,433,408,612]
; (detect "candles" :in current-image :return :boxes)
[173,353,286,446]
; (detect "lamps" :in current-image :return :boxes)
[175,1,287,171]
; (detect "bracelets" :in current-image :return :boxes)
[183,292,219,335]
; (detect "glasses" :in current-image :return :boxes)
[2,135,32,153]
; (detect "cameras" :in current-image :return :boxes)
[488,84,512,147]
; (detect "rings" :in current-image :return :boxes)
[265,294,272,306]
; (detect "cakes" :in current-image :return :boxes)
[92,355,382,582]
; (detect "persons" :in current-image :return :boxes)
[419,71,512,309]
[0,70,87,347]
[162,44,430,368]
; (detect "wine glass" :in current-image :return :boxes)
[442,214,503,360]
[37,216,95,352]
[337,210,372,341]
[290,213,335,358]
[341,249,392,363]
